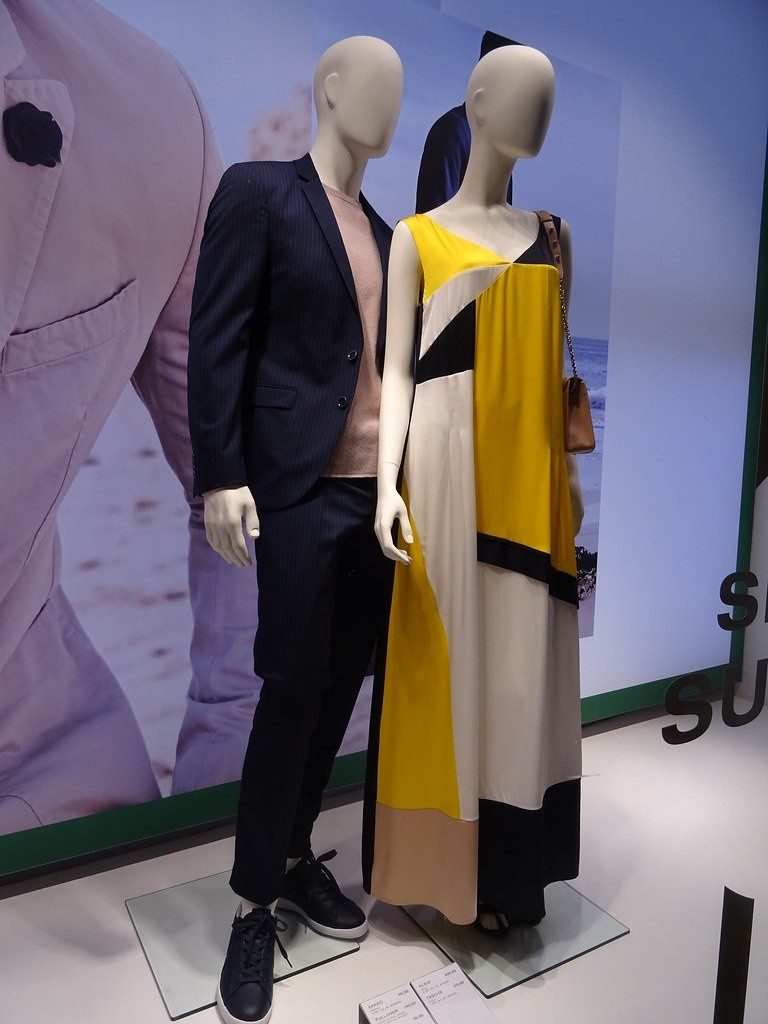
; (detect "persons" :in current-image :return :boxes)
[188,34,423,1024]
[362,44,586,934]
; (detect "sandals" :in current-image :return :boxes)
[471,904,514,938]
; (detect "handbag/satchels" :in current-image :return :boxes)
[532,206,597,454]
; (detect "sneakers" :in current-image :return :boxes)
[214,899,279,1024]
[272,849,370,941]
